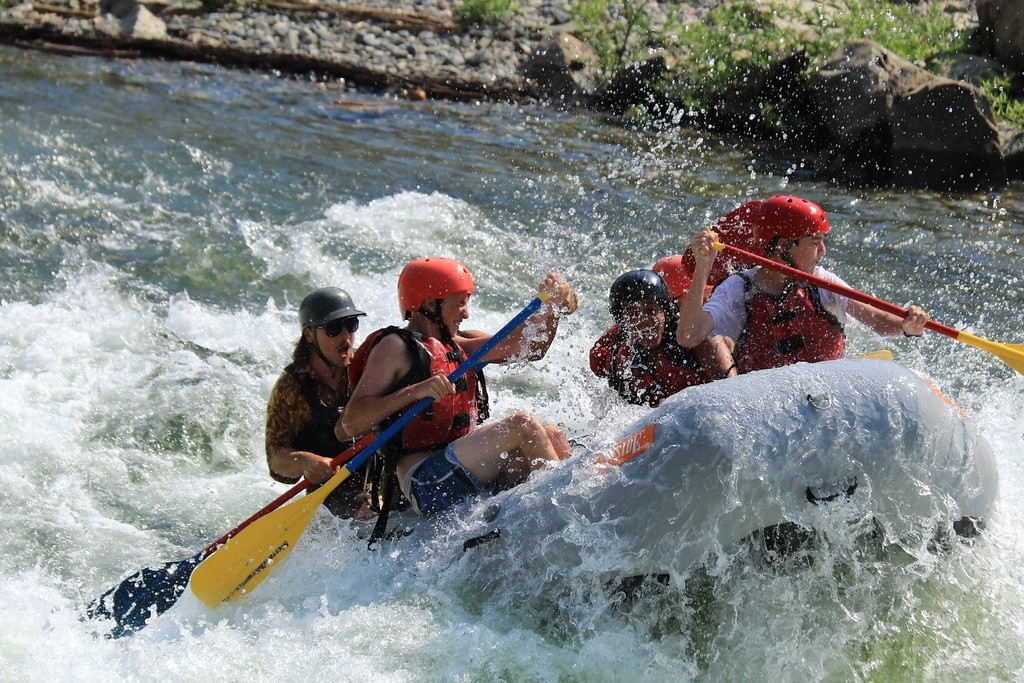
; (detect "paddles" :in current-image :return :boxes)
[80,432,376,641]
[711,242,1024,375]
[188,285,558,602]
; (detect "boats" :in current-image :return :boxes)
[468,357,998,610]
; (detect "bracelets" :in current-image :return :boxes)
[903,331,922,340]
[561,294,579,315]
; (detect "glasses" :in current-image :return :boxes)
[312,316,359,338]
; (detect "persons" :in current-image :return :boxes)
[265,257,578,520]
[590,191,927,405]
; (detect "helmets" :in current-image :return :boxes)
[398,257,475,322]
[608,269,669,325]
[299,287,366,333]
[753,195,829,261]
[653,254,697,298]
